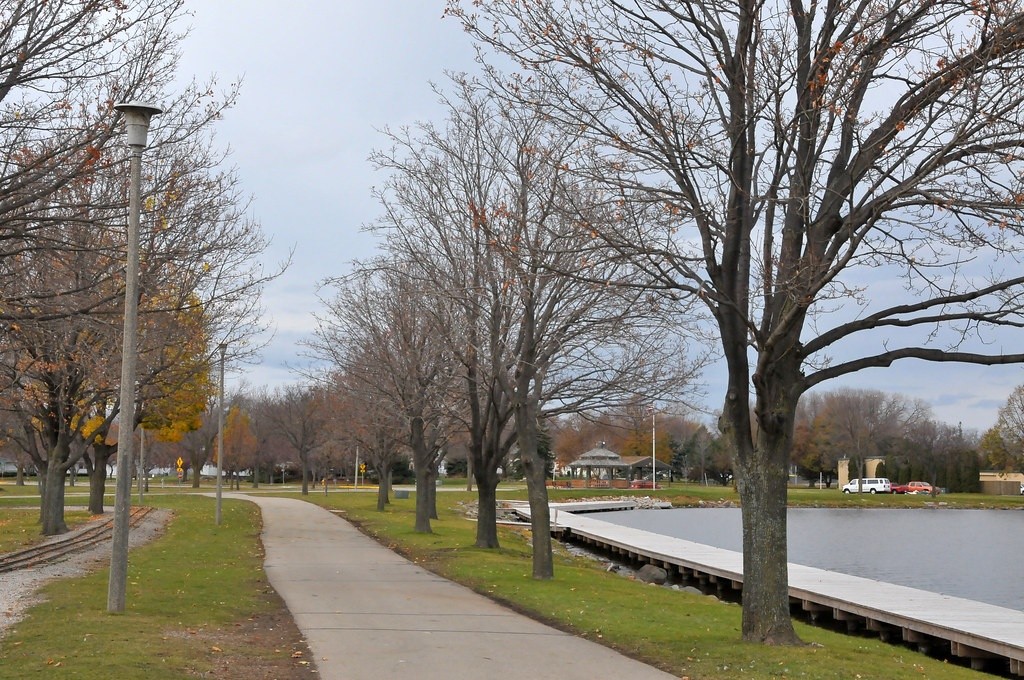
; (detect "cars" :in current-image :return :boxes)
[889,482,909,494]
[905,481,941,495]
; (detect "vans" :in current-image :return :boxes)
[842,477,891,495]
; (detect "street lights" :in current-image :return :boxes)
[106,100,166,611]
[215,342,230,526]
